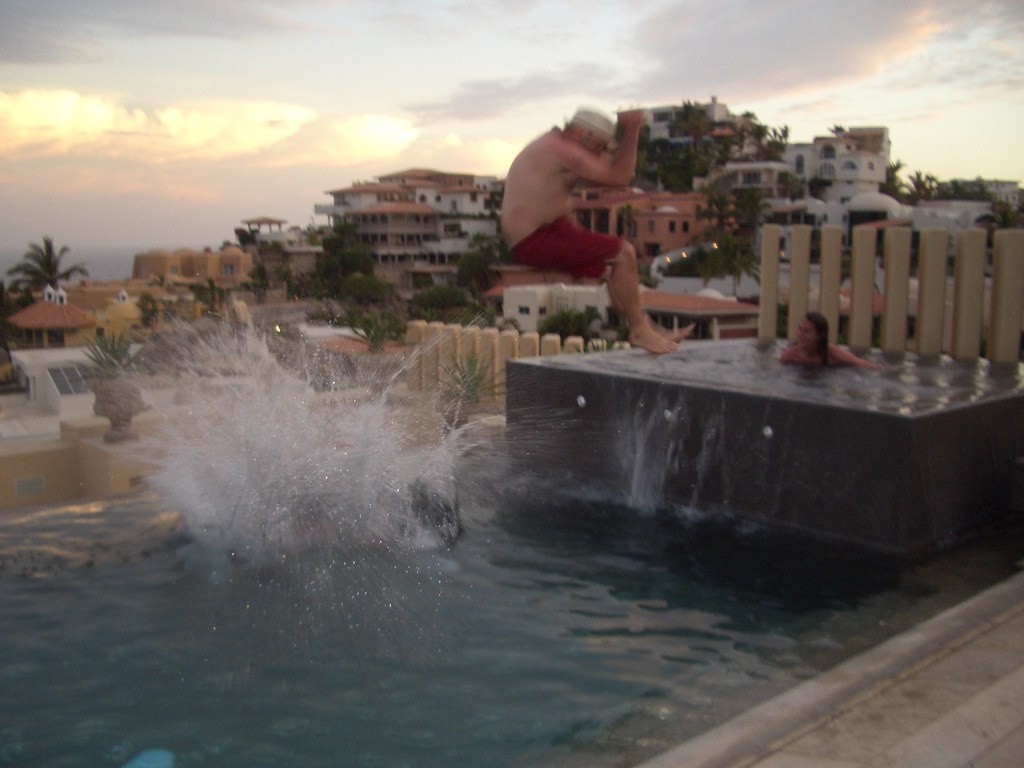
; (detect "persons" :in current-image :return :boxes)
[783,313,869,365]
[501,110,695,353]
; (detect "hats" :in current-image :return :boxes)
[566,110,618,146]
